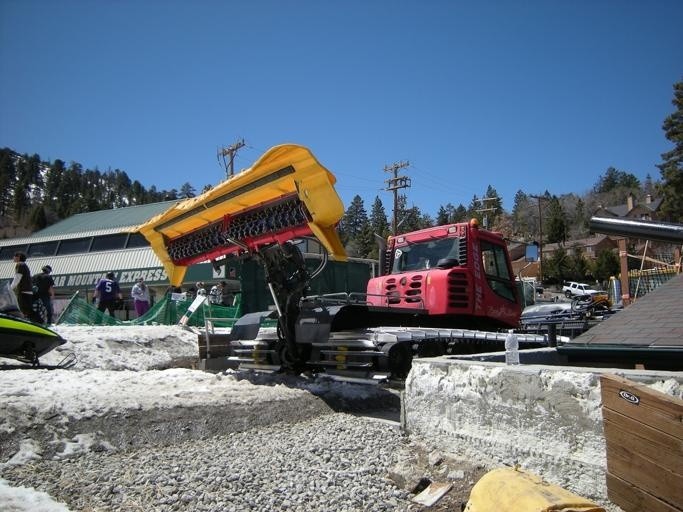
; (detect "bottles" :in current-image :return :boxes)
[505,329,519,365]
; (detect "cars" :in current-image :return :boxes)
[532,280,543,294]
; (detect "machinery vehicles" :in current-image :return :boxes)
[122,144,572,391]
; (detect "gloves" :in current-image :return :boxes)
[92,296,97,304]
[119,299,124,305]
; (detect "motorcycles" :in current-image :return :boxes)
[0,311,77,370]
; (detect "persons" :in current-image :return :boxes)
[11,252,44,324]
[93,272,123,324]
[131,277,152,325]
[165,281,226,306]
[31,266,55,325]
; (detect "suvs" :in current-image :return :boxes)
[560,280,599,302]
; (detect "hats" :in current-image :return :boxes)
[106,271,114,278]
